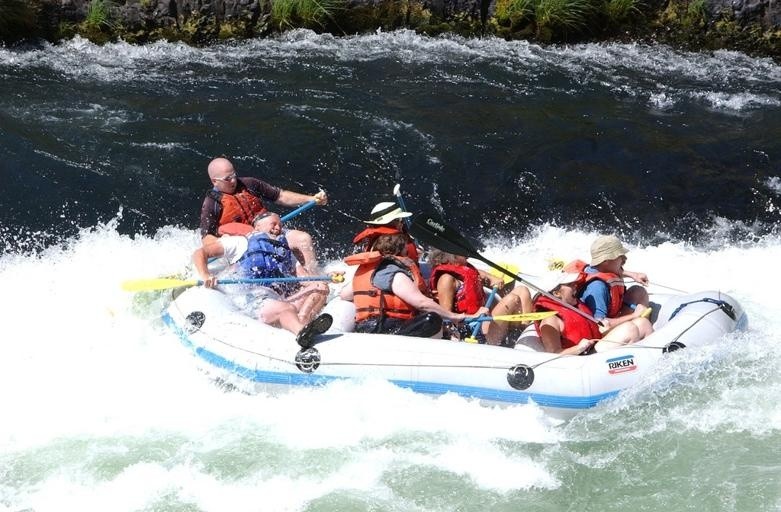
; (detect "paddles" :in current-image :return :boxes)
[547,257,653,318]
[123,278,345,294]
[134,200,320,306]
[465,265,518,344]
[440,312,558,322]
[406,209,605,328]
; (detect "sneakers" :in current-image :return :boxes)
[296,313,333,348]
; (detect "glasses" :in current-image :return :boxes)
[215,171,237,182]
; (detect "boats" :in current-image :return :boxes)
[160,259,750,422]
[158,285,749,422]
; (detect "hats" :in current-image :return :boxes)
[590,234,629,266]
[545,270,581,292]
[362,201,414,225]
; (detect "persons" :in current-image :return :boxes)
[344,234,490,337]
[200,158,328,266]
[579,236,650,330]
[352,201,419,262]
[531,269,654,355]
[192,212,346,349]
[429,251,531,345]
